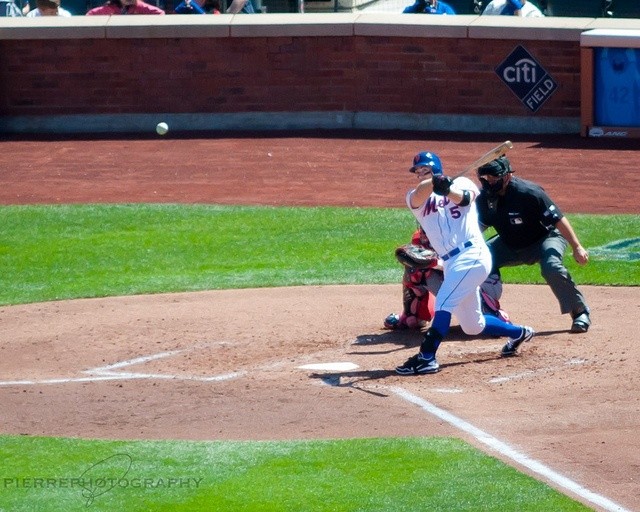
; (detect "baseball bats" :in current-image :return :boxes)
[449,140,513,181]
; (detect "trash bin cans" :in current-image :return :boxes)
[593,46,640,126]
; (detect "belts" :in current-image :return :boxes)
[442,241,472,261]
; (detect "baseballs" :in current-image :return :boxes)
[155,121,170,136]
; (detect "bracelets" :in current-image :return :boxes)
[454,190,470,207]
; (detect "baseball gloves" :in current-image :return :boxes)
[396,244,439,272]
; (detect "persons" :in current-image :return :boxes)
[85,0,166,17]
[475,153,591,333]
[25,0,72,17]
[186,0,255,14]
[384,222,513,332]
[481,0,546,17]
[401,0,457,15]
[395,151,535,375]
[0,0,30,17]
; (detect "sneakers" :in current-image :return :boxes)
[571,318,587,331]
[385,312,428,328]
[395,352,439,374]
[501,327,535,355]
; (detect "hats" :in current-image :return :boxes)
[409,152,442,176]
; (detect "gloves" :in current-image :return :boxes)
[433,188,450,196]
[432,176,454,188]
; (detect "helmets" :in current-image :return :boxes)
[478,157,511,203]
